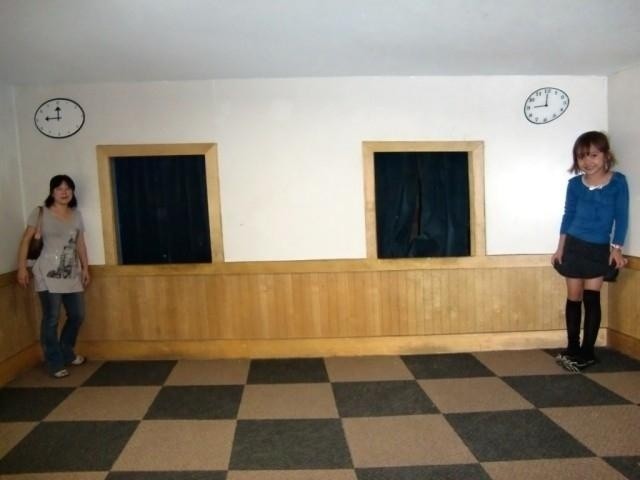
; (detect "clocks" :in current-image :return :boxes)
[524,87,570,125]
[34,97,85,140]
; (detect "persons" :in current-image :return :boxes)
[17,174,92,379]
[549,128,631,373]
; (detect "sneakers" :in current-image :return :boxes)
[555,350,595,371]
[72,355,85,365]
[54,368,69,378]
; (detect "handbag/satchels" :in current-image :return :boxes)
[27,207,44,259]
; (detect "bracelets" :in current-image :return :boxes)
[610,243,624,251]
